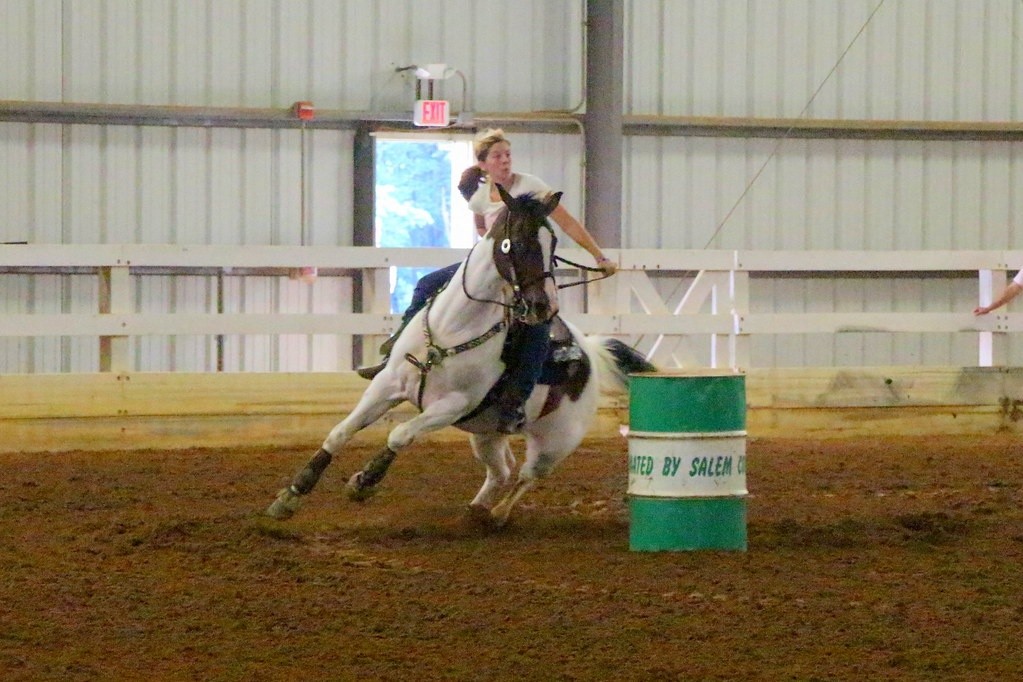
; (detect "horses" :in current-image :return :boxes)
[267,182,658,535]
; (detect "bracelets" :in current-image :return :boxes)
[597,257,609,263]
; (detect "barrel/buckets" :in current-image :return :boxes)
[625,372,749,554]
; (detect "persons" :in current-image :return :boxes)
[358,128,617,381]
[972,269,1023,316]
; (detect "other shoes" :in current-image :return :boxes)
[497,412,526,433]
[357,362,385,380]
